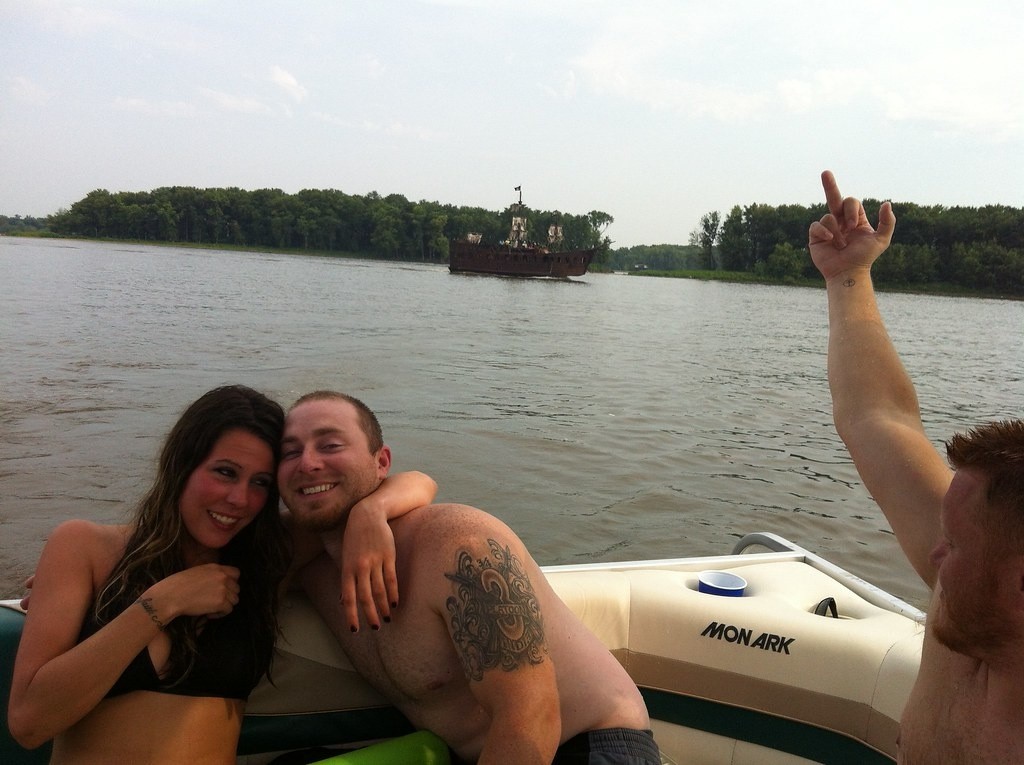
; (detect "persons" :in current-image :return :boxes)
[810,172,1023,765]
[503,238,547,253]
[21,390,659,765]
[8,383,439,765]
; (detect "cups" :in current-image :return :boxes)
[698,571,747,597]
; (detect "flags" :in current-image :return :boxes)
[514,186,520,191]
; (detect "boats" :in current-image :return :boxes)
[448,184,615,276]
